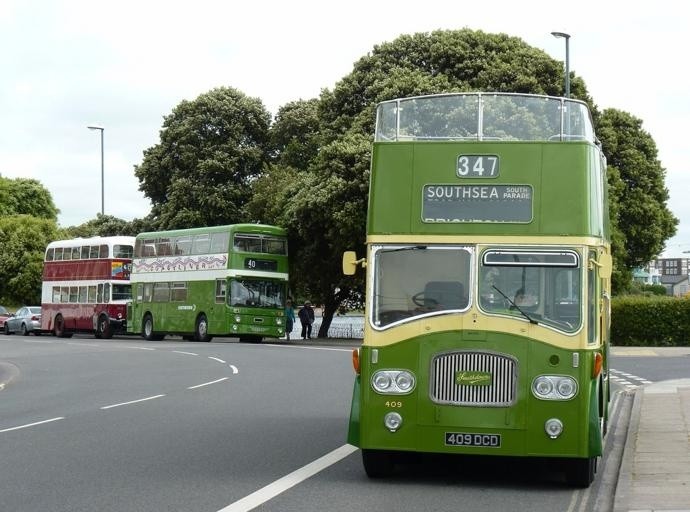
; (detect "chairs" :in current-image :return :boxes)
[420,280,466,313]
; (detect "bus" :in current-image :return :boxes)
[340,87,616,489]
[39,234,137,340]
[125,222,291,342]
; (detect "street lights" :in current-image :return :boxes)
[87,126,105,215]
[550,31,572,142]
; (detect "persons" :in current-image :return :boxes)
[285,298,296,342]
[298,300,317,340]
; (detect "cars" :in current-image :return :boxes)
[3,305,53,336]
[0,306,12,330]
